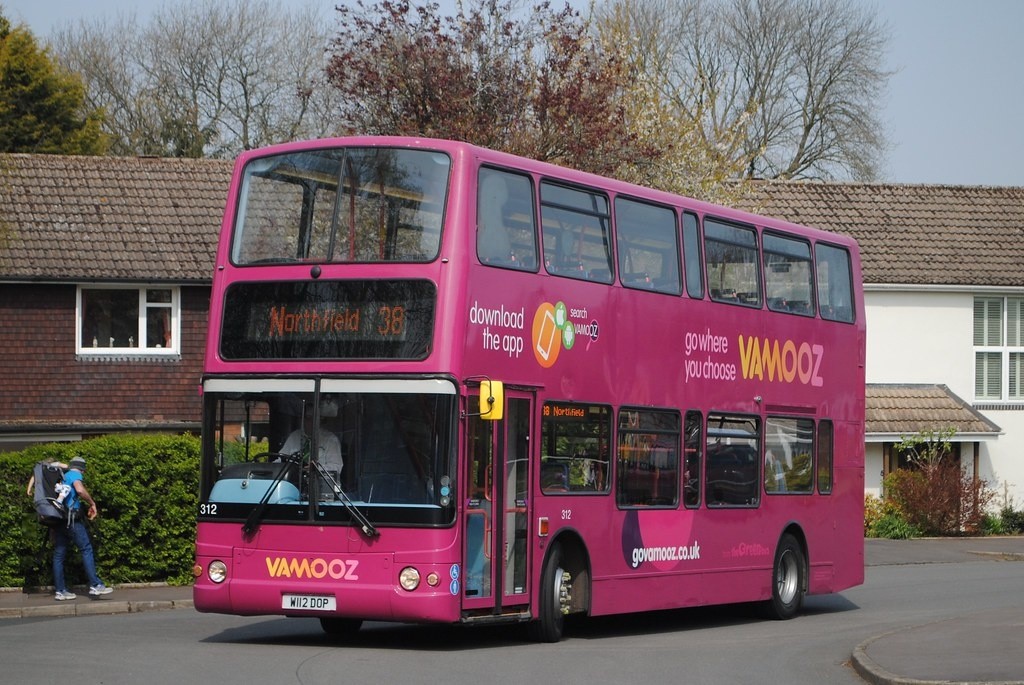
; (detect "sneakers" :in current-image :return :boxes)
[55,590,76,600]
[89,583,114,595]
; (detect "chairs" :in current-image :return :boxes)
[479,254,853,323]
[540,462,571,493]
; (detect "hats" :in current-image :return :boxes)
[69,456,86,471]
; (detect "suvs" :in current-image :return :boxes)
[688,419,759,505]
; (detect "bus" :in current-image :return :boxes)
[192,135,867,643]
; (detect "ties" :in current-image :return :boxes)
[303,439,310,465]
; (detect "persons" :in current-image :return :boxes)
[27,457,68,496]
[53,455,114,601]
[273,404,343,492]
